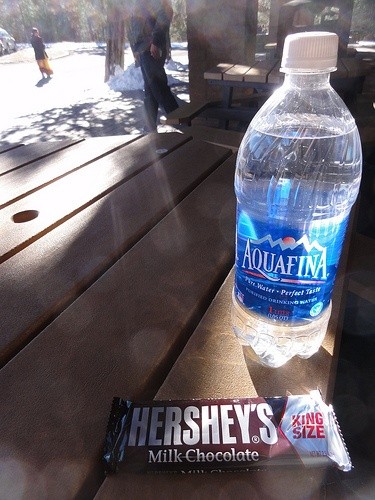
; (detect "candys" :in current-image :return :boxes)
[100,392,354,472]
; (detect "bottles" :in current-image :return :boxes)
[229,30,363,370]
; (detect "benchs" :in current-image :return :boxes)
[156,96,375,156]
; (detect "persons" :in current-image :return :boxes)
[119,0,186,134]
[31,28,54,79]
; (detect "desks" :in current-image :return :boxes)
[0,133,375,500]
[204,60,367,134]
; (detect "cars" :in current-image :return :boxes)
[0,28,17,57]
